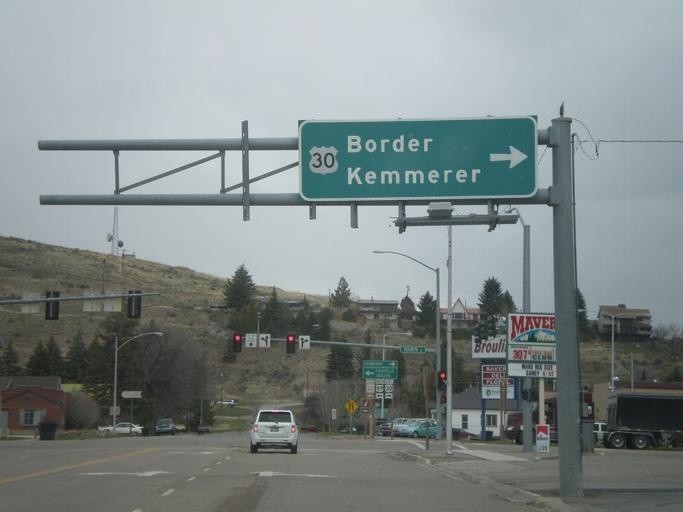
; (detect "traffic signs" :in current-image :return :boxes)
[362,360,398,380]
[295,112,539,205]
[399,343,426,353]
[120,390,143,399]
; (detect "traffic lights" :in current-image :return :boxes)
[471,321,482,344]
[285,333,296,354]
[232,333,242,353]
[487,319,496,338]
[477,321,488,341]
[437,370,445,390]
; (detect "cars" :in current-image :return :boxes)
[392,418,437,439]
[299,424,320,433]
[95,420,143,434]
[592,422,608,439]
[337,413,411,436]
[196,422,211,434]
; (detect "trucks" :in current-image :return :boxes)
[502,391,682,450]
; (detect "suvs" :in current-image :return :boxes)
[154,417,176,436]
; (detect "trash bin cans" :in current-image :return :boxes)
[38,422,56,440]
[580,420,594,453]
[481,431,493,441]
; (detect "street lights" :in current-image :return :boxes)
[111,330,163,425]
[371,247,443,425]
[504,207,534,451]
[578,307,651,395]
[380,331,413,418]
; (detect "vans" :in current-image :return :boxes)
[246,407,300,455]
[215,398,237,407]
